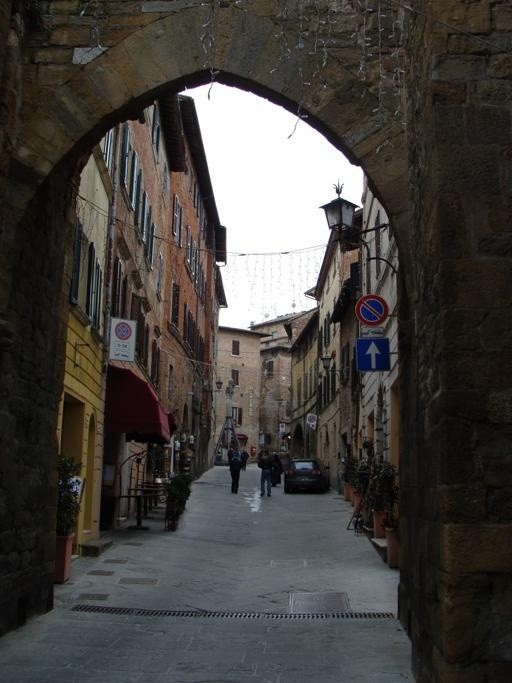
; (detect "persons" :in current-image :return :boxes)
[227,443,290,497]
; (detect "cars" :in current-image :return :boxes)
[284,457,330,494]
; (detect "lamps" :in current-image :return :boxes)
[319,179,387,255]
[321,350,342,376]
[215,376,225,392]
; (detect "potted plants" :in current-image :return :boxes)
[54,445,85,585]
[341,455,401,569]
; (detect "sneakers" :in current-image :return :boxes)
[260,493,271,497]
